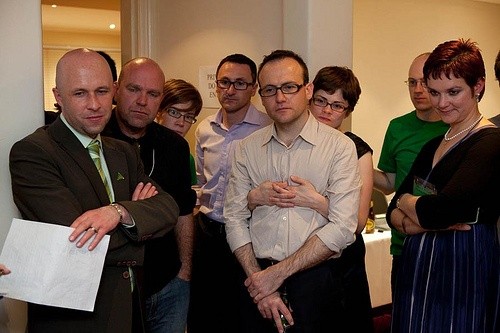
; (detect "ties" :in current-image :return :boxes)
[87,141,114,204]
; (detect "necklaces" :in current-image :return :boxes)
[444,115,482,141]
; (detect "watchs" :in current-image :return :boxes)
[396,193,405,211]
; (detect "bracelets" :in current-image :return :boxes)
[401,215,408,234]
[110,203,123,223]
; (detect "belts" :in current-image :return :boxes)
[199,214,225,236]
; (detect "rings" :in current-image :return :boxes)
[89,227,96,232]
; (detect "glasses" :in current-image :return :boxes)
[405,79,427,88]
[258,82,305,97]
[311,95,350,113]
[215,80,253,90]
[165,108,197,124]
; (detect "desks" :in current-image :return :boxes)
[363,229,397,307]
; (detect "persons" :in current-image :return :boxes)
[386,38,500,333]
[103,57,197,333]
[223,49,361,333]
[247,65,376,333]
[192,54,276,333]
[373,52,452,333]
[156,79,204,186]
[9,48,180,333]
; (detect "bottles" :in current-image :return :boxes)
[365,199,375,233]
